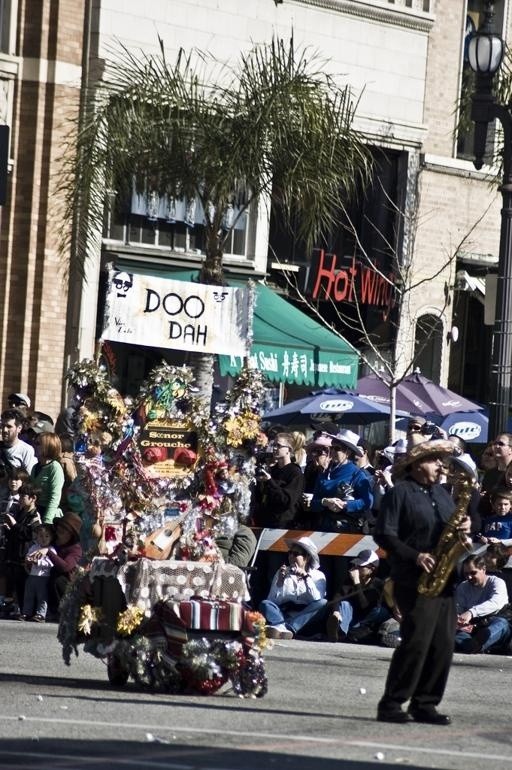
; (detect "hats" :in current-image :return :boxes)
[284,537,320,569]
[349,550,379,568]
[386,439,407,453]
[447,453,478,477]
[8,393,30,407]
[52,512,82,542]
[408,416,426,425]
[322,428,364,458]
[375,447,394,464]
[389,423,462,474]
[307,436,332,447]
[27,421,54,434]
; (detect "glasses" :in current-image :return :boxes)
[494,441,509,446]
[288,550,303,556]
[273,443,288,448]
[463,571,480,575]
[331,446,343,450]
[316,450,326,456]
[408,425,421,430]
[8,401,24,407]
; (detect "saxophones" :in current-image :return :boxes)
[416,471,476,596]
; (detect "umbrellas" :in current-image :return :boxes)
[261,386,412,426]
[355,370,436,414]
[398,366,484,416]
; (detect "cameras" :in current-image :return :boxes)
[421,420,435,434]
[255,463,271,477]
[289,565,296,573]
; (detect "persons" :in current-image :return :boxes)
[484,542,509,579]
[328,549,390,643]
[478,491,512,546]
[31,432,64,525]
[2,483,41,605]
[251,431,306,528]
[437,426,477,470]
[407,416,425,434]
[372,447,395,517]
[0,469,31,550]
[8,392,30,412]
[303,437,333,510]
[372,434,471,726]
[441,451,481,541]
[59,435,76,483]
[17,525,55,623]
[377,438,406,490]
[481,442,497,469]
[29,512,83,607]
[356,441,374,474]
[479,432,512,497]
[454,553,508,653]
[258,535,327,639]
[0,409,37,498]
[504,459,512,490]
[310,428,374,535]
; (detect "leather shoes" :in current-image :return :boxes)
[407,705,451,725]
[377,707,414,723]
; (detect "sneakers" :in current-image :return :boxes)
[264,625,292,639]
[327,614,340,642]
[382,634,401,648]
[33,615,46,623]
[19,614,29,620]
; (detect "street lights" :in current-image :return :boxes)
[466,2,512,439]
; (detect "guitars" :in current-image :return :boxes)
[143,499,197,561]
